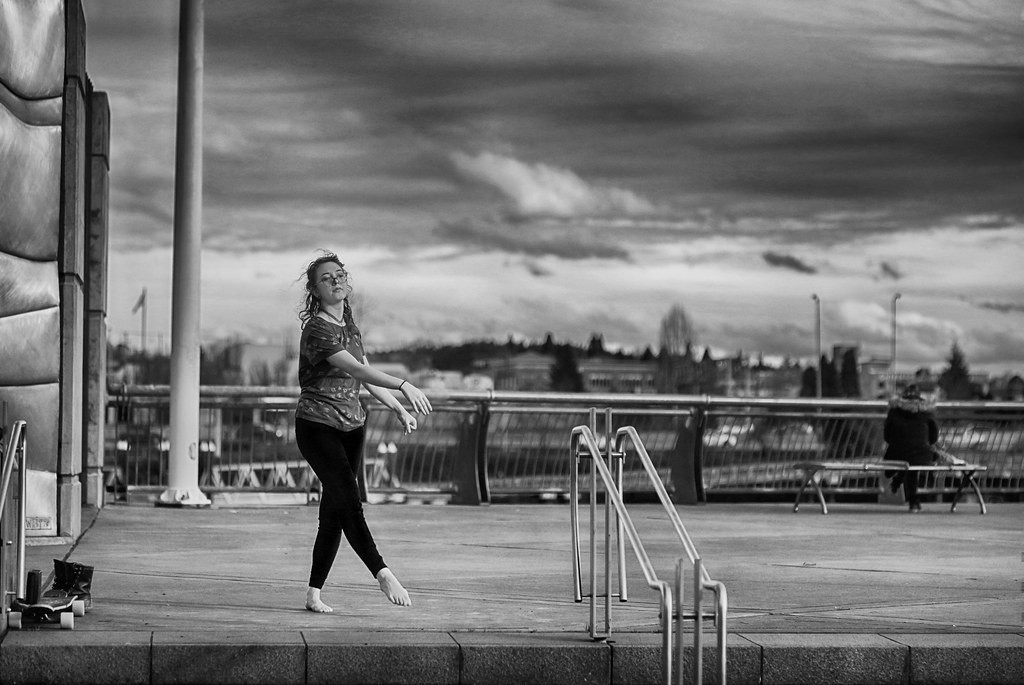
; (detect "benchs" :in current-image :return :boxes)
[794,460,988,515]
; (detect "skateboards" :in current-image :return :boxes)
[8,590,85,630]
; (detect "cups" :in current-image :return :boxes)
[26,570,42,603]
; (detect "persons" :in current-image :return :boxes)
[294,255,432,614]
[881,384,939,513]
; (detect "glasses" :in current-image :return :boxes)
[314,273,346,287]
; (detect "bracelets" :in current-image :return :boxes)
[398,380,407,390]
